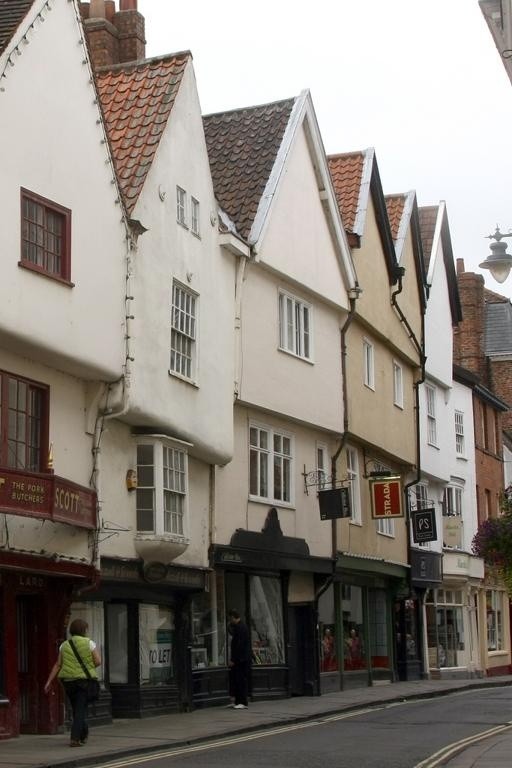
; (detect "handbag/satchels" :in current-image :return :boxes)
[85,679,102,702]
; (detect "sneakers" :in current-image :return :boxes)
[224,703,248,710]
[69,731,89,748]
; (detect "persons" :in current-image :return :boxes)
[225,608,255,709]
[44,619,101,747]
